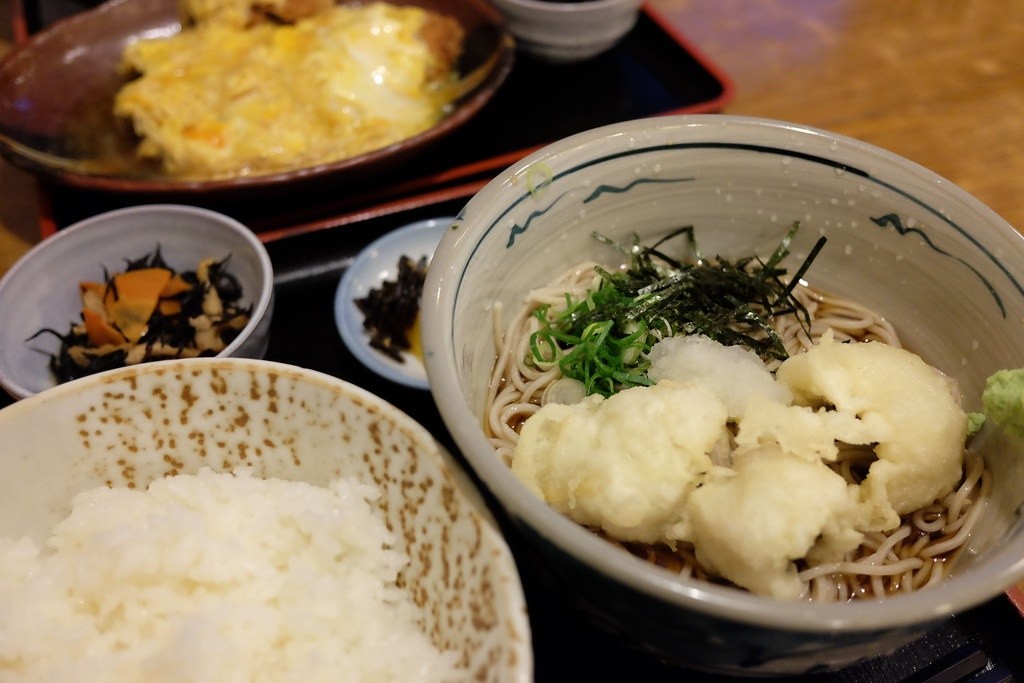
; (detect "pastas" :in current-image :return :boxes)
[490,265,990,606]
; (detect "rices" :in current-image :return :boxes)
[1,466,469,683]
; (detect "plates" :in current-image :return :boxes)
[335,217,457,388]
[0,0,515,191]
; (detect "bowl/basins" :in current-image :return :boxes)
[489,0,642,59]
[420,113,1024,631]
[0,358,533,683]
[0,205,274,400]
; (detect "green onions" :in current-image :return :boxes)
[528,274,653,390]
